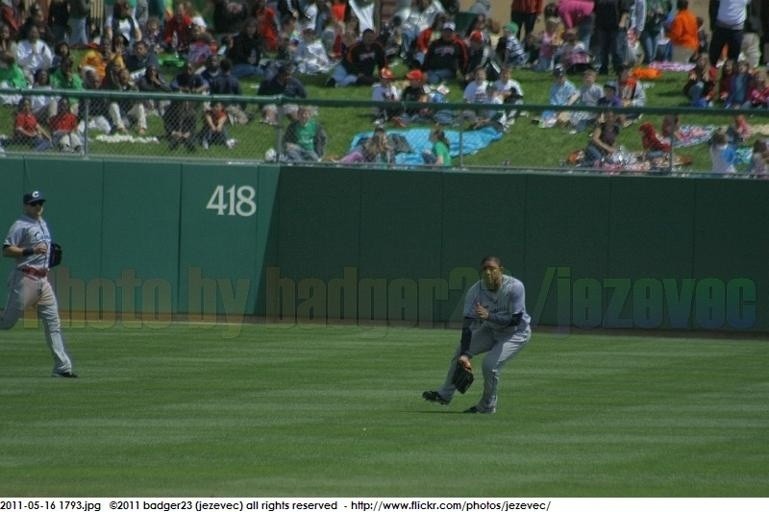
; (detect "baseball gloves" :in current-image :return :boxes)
[47,243,62,268]
[452,359,474,394]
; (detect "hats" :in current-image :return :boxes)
[379,67,423,82]
[553,63,637,91]
[22,189,45,205]
[434,12,521,45]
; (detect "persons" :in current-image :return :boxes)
[422,255,533,414]
[1,188,82,379]
[1,0,767,176]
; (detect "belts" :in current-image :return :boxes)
[16,265,50,279]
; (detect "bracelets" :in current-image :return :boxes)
[22,246,34,257]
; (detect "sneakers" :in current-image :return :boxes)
[463,405,480,414]
[423,389,451,406]
[49,366,80,379]
